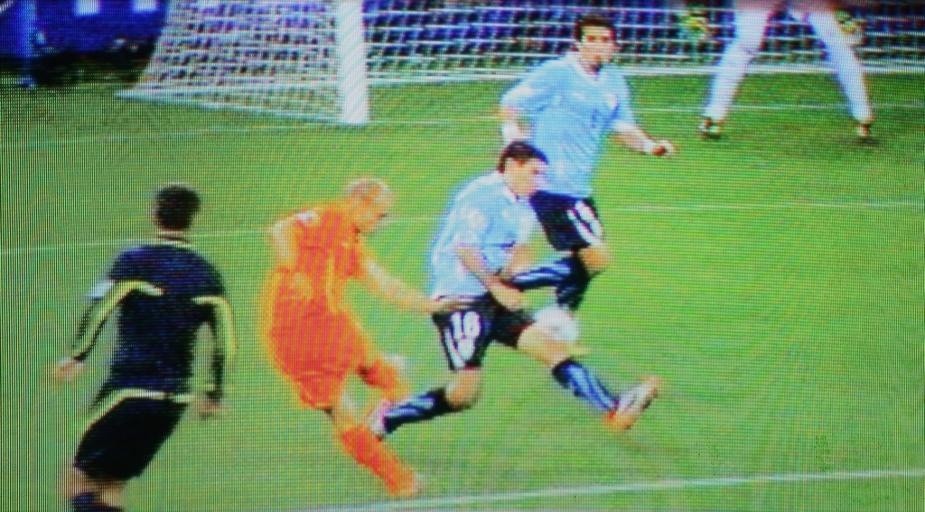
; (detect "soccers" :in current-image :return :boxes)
[535,307,579,343]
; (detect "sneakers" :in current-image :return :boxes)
[608,374,662,439]
[855,119,876,145]
[698,116,726,142]
[366,400,395,442]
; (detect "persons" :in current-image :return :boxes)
[40,186,229,512]
[257,176,478,499]
[363,140,667,443]
[695,1,879,144]
[499,12,675,358]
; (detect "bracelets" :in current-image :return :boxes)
[500,122,522,145]
[639,138,656,158]
